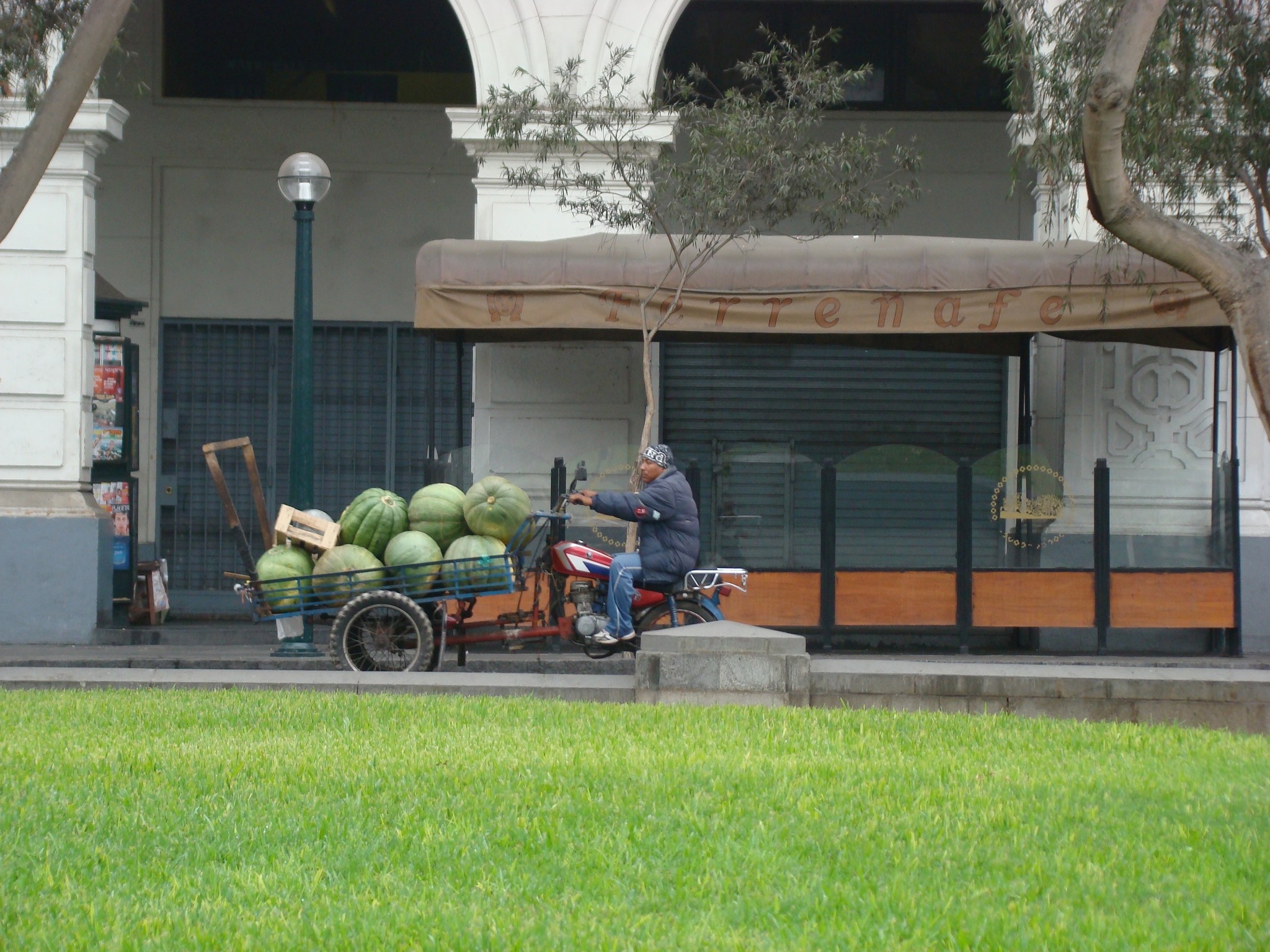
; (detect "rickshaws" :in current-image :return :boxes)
[204,434,747,674]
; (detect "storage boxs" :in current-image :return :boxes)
[273,504,341,560]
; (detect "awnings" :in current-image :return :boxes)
[413,232,1262,335]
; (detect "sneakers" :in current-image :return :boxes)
[592,629,636,643]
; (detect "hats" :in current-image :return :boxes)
[642,444,674,468]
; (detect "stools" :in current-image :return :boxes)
[129,560,169,626]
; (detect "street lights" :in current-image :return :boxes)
[272,148,331,657]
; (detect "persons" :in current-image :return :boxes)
[570,444,699,643]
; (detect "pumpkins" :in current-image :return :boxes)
[254,476,535,615]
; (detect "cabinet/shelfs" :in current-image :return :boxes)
[91,336,139,602]
